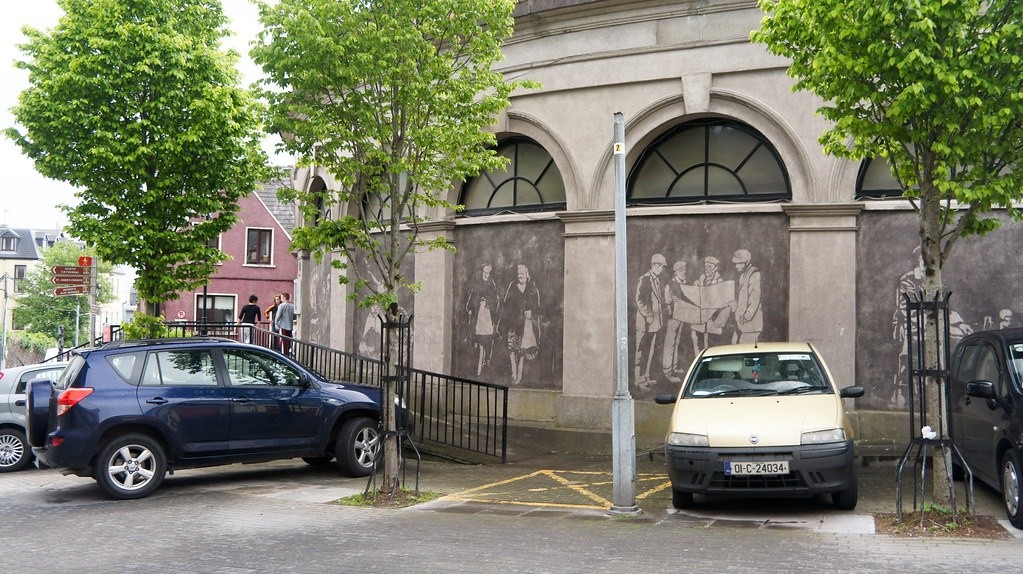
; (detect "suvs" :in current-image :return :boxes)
[27,336,414,500]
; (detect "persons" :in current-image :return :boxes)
[238,294,262,343]
[264,292,295,356]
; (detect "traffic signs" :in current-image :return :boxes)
[52,286,92,297]
[51,276,91,286]
[50,265,91,275]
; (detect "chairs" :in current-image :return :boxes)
[717,371,742,380]
[779,360,812,386]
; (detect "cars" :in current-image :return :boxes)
[654,341,865,510]
[0,360,70,473]
[943,325,1023,530]
